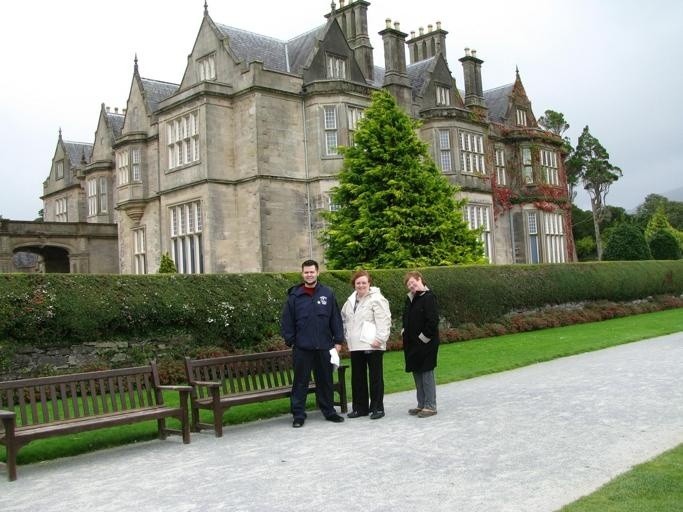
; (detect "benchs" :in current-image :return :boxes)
[183,346,350,438]
[0,359,194,482]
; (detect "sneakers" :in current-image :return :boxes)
[409,407,437,418]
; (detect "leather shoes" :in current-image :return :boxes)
[371,411,385,419]
[348,410,369,418]
[326,414,344,422]
[293,418,304,427]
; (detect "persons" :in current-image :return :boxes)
[339,270,392,420]
[280,258,345,427]
[399,270,441,418]
